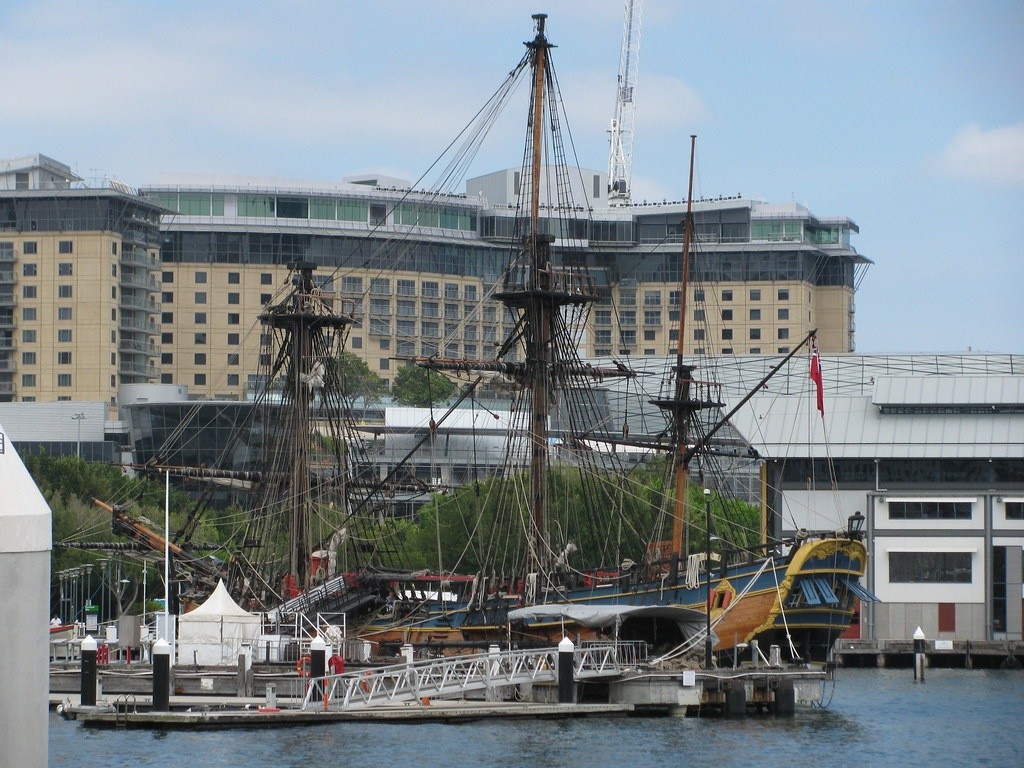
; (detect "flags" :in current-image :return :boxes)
[810,333,824,419]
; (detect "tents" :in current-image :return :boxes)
[178,578,261,665]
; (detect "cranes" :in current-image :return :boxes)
[606,0,642,209]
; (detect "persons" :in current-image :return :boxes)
[50,615,61,625]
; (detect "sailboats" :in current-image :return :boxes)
[49,15,884,712]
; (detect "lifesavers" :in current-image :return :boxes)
[296,656,314,677]
[362,671,374,693]
